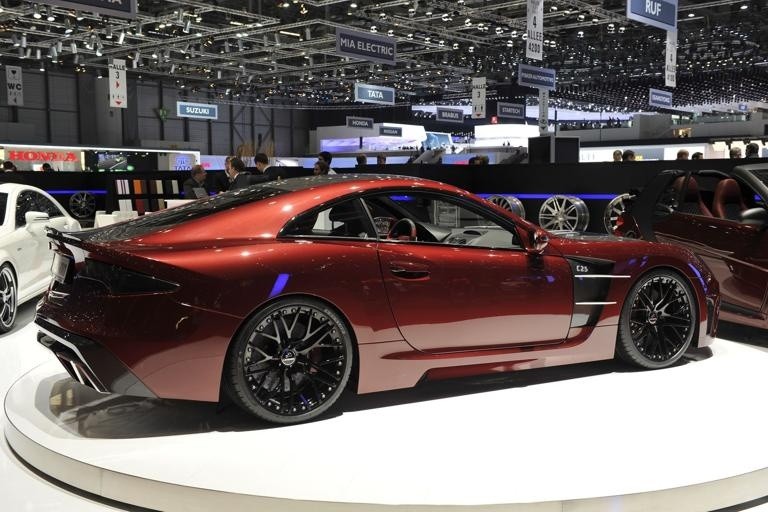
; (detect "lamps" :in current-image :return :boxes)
[10,0,382,105]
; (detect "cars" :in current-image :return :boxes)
[1,182,85,334]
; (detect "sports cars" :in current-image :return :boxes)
[31,170,723,429]
[622,155,767,335]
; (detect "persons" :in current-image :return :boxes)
[729,147,742,158]
[213,156,237,193]
[312,160,330,176]
[227,158,264,193]
[677,149,689,160]
[371,155,395,173]
[692,152,704,159]
[622,150,636,161]
[183,166,208,198]
[353,152,373,172]
[3,161,14,172]
[318,151,338,175]
[745,143,760,158]
[42,163,54,170]
[254,153,289,182]
[613,149,623,162]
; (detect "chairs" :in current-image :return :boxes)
[331,201,375,239]
[673,175,749,223]
